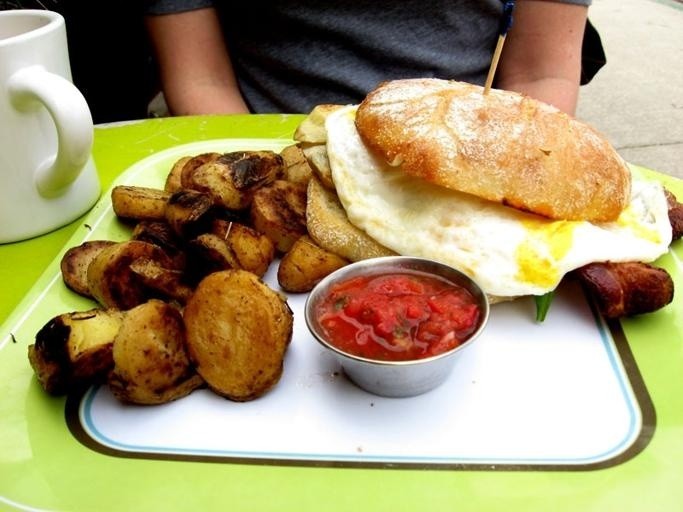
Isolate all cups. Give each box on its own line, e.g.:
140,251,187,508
1,8,103,246
302,255,489,398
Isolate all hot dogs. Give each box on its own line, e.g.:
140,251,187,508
293,76,683,320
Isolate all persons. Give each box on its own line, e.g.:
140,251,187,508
141,1,590,118
